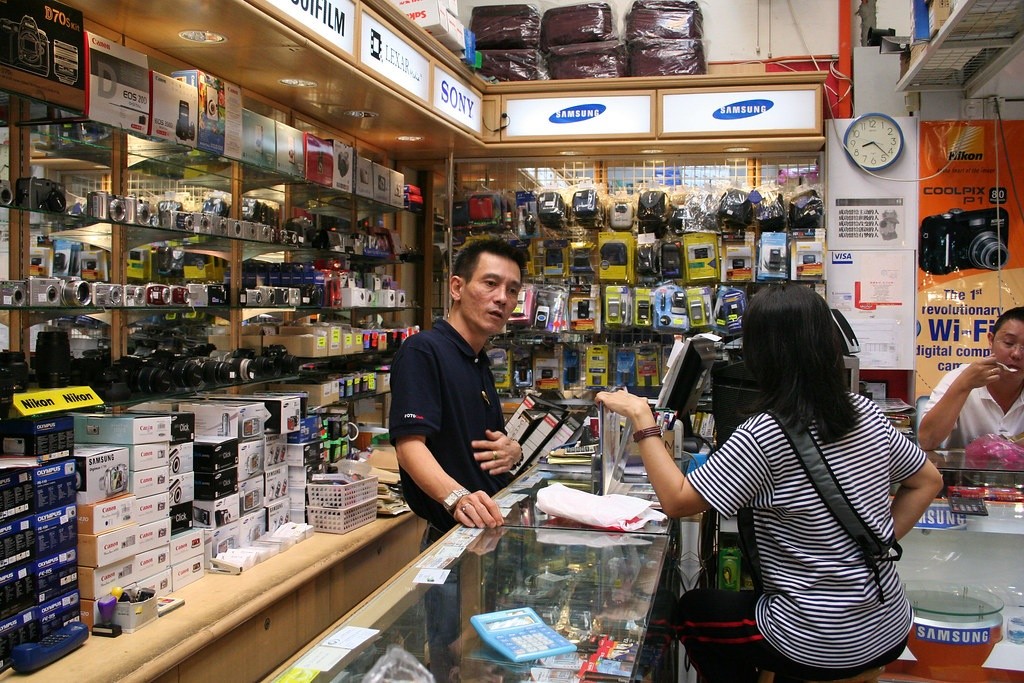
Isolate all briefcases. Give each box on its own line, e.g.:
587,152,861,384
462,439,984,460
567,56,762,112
469,3,542,80
626,0,706,77
542,2,627,78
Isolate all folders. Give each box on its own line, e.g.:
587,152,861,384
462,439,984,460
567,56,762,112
505,393,584,479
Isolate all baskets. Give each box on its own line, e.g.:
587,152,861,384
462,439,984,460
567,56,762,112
305,473,379,535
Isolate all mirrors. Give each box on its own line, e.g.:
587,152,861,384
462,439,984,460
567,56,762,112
0,143,314,350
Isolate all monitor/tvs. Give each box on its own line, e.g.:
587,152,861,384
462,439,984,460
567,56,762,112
662,336,718,454
713,352,859,451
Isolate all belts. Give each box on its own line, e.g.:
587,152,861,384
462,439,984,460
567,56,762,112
425,524,444,542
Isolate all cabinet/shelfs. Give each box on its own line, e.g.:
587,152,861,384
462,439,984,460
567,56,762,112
451,82,825,424
263,455,691,683
0,0,487,683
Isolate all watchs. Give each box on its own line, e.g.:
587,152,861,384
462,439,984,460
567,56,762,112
442,488,472,516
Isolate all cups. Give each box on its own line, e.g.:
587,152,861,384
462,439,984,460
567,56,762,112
661,430,675,461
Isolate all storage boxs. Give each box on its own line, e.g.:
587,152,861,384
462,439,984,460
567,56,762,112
341,286,405,308
171,69,425,212
209,324,262,355
391,0,482,72
899,0,950,78
263,321,419,358
0,370,391,675
717,544,742,591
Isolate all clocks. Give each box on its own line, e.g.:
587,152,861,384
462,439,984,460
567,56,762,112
843,113,904,172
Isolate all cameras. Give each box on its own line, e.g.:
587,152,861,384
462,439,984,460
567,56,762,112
176,100,195,142
361,168,370,185
206,87,218,121
287,136,296,165
98,463,129,495
377,175,387,192
214,509,232,527
919,206,1010,275
192,507,211,525
253,125,264,155
0,175,328,403
86,425,99,435
3,437,24,454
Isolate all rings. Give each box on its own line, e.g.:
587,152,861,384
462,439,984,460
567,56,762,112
463,508,466,513
990,370,995,375
492,450,498,460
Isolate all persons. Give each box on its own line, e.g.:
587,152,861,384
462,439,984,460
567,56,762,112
592,285,944,683
388,235,525,683
917,307,1024,489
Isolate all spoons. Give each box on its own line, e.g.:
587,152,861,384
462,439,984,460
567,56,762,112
996,361,1018,373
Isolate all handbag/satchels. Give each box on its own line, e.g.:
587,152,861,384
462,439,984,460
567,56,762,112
717,188,755,231
637,190,670,240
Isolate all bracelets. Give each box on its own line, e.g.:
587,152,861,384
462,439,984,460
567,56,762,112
508,437,524,470
633,425,662,443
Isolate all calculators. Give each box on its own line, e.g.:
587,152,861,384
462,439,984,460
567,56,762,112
470,607,577,661
948,495,989,515
